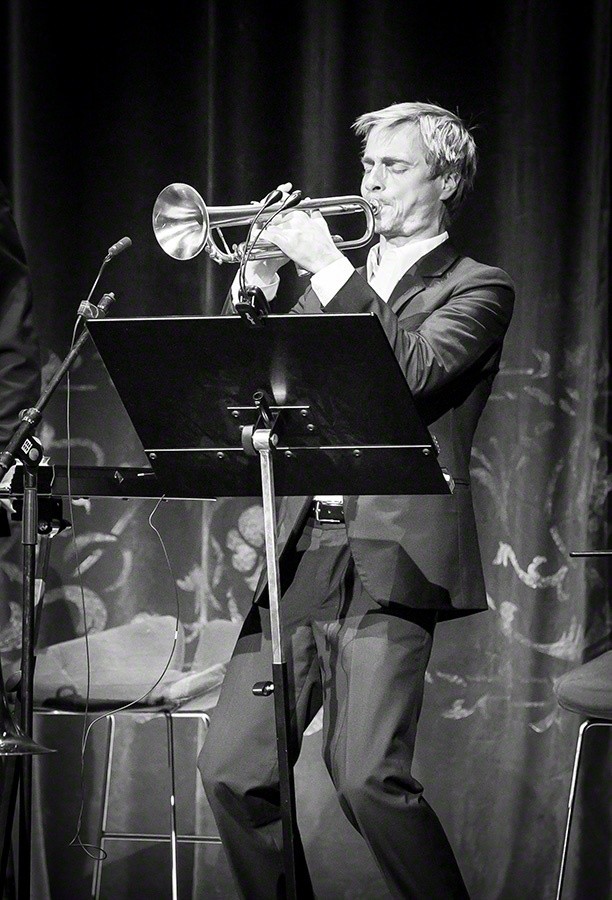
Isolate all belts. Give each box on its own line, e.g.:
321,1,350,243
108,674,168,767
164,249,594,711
306,500,347,523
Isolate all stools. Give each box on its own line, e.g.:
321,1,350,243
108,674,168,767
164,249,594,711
33,617,244,900
554,649,612,900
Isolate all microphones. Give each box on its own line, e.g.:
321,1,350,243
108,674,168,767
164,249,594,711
108,236,132,257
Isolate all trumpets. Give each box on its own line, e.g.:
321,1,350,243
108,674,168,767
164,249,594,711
152,182,383,266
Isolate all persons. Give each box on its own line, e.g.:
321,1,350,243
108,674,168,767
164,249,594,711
1,255,42,451
196,102,517,900
